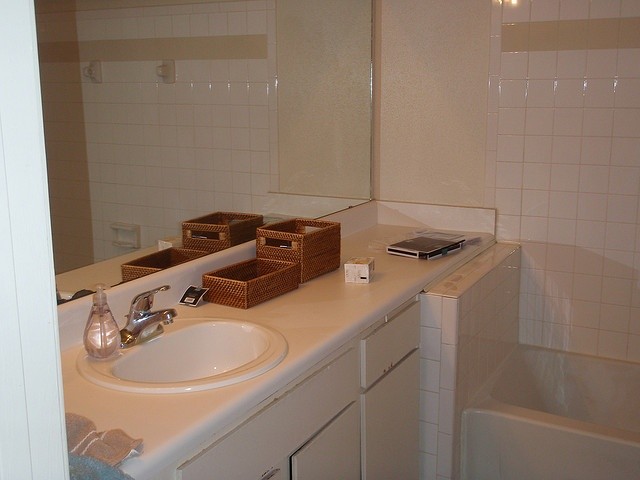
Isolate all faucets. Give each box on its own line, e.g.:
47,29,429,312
121,284,177,348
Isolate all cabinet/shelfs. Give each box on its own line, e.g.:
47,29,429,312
358,296,421,480
176,345,359,480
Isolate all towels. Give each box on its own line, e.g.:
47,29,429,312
68,455,135,480
66,413,143,468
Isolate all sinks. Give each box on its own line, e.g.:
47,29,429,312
111,321,271,383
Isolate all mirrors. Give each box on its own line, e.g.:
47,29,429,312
34,0,373,304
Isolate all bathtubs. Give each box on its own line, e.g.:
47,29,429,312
461,342,640,480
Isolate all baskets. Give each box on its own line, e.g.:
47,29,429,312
181,211,265,252
203,258,301,310
121,247,208,283
255,218,341,282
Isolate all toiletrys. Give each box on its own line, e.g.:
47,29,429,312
83,283,121,360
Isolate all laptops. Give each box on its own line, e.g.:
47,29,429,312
387,237,461,260
